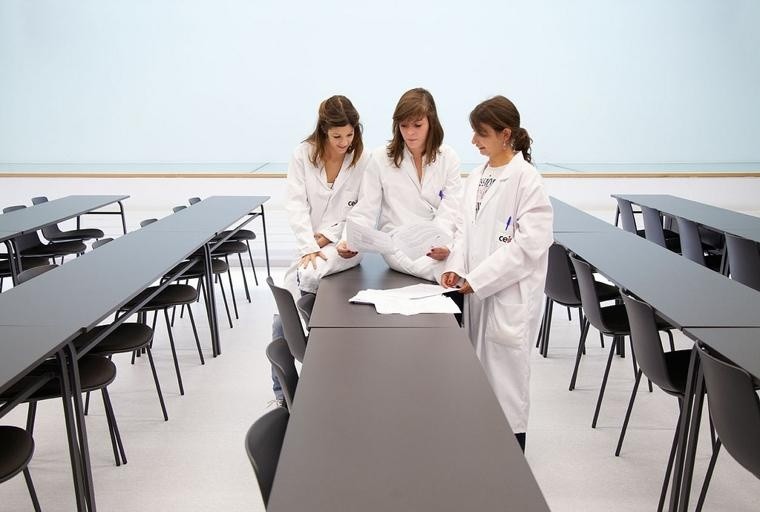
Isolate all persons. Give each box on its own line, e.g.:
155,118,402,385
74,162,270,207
336,88,468,327
442,96,555,455
269,95,371,410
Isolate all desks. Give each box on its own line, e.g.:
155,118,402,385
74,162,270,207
550,196,760,512
265,250,553,512
2,195,272,437
1,188,131,241
608,194,760,244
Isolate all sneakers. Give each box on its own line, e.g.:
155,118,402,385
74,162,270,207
265,399,287,409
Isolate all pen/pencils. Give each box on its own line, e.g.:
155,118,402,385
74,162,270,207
438,190,443,202
503,215,512,232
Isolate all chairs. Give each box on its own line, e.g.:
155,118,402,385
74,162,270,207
567,252,676,429
3,204,86,276
266,276,309,363
11,263,170,424
540,242,608,358
265,336,299,413
90,237,205,396
674,216,705,267
0,194,270,512
640,205,667,249
725,232,760,292
616,197,638,235
297,293,316,325
695,339,760,497
170,206,253,303
244,406,291,511
614,285,702,510
30,196,105,259
188,196,272,279
138,218,239,329
